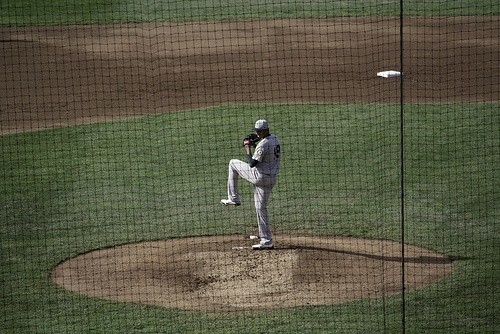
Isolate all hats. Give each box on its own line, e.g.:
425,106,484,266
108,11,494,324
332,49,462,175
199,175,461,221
251,119,268,130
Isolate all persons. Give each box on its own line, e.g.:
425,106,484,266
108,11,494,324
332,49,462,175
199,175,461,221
220,119,280,249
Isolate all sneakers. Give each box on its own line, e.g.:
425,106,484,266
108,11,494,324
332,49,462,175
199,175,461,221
252,244,274,250
220,199,241,206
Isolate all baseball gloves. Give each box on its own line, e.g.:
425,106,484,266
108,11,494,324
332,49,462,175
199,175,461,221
242,133,260,147
